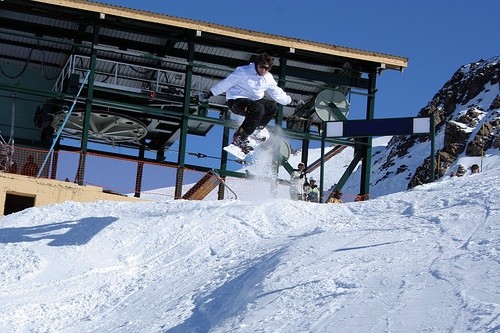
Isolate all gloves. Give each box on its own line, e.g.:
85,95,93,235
287,98,305,107
198,90,213,102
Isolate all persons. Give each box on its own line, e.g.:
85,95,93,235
450,171,456,176
327,188,343,203
0,150,18,172
471,163,480,173
198,51,307,155
310,176,319,202
20,155,41,176
302,181,311,201
354,192,366,201
289,161,306,201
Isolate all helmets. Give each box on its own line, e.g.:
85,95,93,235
309,176,317,183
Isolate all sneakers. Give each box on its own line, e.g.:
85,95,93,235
251,128,266,142
233,130,254,153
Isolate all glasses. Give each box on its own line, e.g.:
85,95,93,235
259,64,271,69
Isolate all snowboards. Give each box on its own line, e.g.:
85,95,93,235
223,127,270,161
